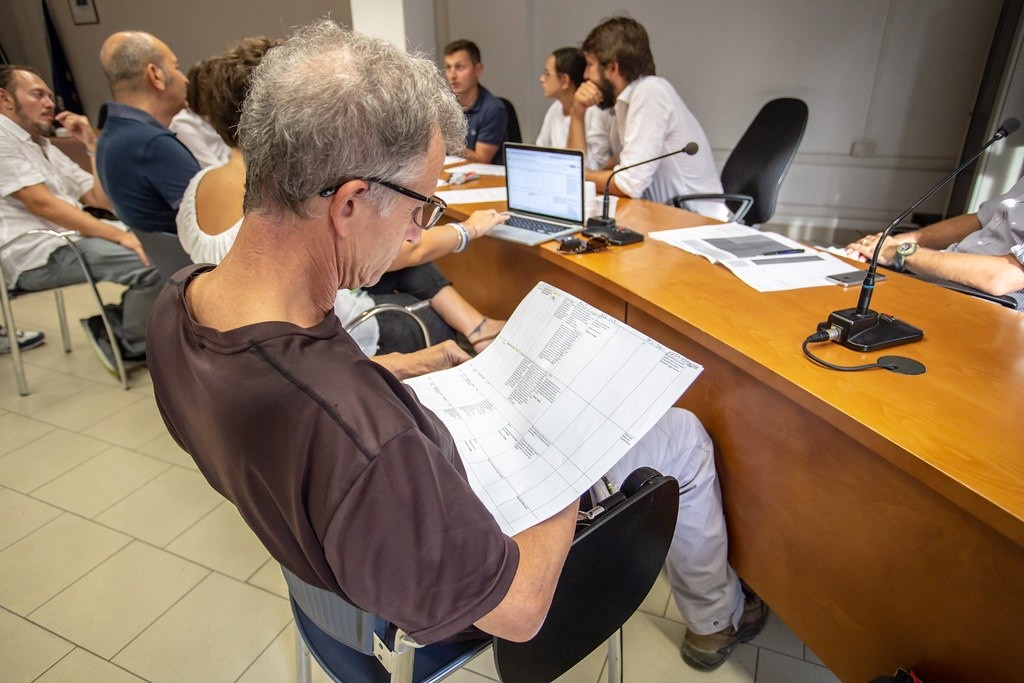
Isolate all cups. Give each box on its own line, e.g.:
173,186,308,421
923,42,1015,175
585,181,596,220
595,195,619,220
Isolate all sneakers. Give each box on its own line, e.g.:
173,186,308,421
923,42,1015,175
79,315,122,376
0,324,45,353
681,576,770,671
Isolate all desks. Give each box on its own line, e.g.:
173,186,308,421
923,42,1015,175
429,149,1024,683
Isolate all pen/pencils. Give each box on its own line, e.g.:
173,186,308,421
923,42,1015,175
764,249,805,255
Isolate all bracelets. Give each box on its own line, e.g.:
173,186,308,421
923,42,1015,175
86,151,96,156
446,222,469,253
467,222,478,238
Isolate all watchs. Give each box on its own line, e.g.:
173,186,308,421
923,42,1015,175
895,242,917,272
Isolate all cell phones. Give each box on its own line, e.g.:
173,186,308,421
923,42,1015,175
826,270,885,288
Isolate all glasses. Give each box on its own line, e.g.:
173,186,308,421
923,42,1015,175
555,235,611,254
537,71,562,77
321,177,449,231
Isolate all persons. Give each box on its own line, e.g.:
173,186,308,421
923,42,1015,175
95,31,203,234
534,47,601,171
0,64,190,376
145,23,764,669
174,38,510,356
443,40,508,163
169,63,232,169
843,177,1024,322
567,16,730,223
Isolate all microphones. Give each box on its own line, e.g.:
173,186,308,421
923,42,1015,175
817,117,1022,353
583,142,698,246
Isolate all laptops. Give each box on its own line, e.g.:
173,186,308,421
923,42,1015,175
483,143,586,246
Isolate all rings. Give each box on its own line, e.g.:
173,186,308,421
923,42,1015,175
491,212,495,216
862,239,866,244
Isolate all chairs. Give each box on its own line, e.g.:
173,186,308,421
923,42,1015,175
673,96,809,227
134,228,435,352
279,470,625,683
0,203,137,397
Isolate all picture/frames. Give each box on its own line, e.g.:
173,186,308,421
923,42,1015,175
67,0,101,26
